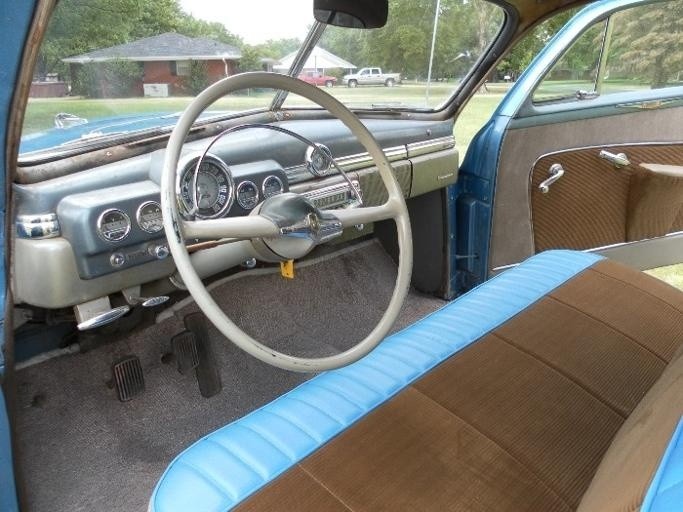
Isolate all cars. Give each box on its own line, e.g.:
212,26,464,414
297,70,337,88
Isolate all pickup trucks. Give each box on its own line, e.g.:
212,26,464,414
342,67,402,88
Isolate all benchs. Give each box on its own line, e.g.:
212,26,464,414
148,248,682,511
